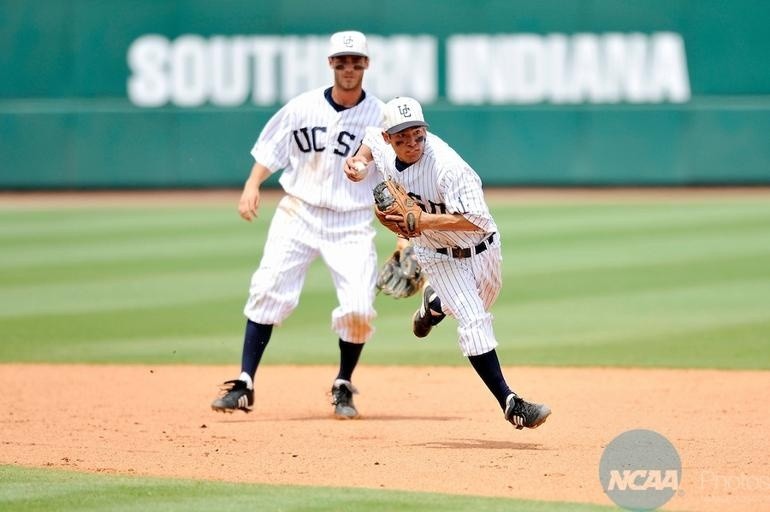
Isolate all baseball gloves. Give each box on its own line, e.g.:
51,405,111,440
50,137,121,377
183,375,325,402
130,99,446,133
376,238,426,299
372,175,422,240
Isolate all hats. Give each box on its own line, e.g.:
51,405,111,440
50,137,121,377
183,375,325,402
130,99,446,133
327,30,369,58
380,96,429,134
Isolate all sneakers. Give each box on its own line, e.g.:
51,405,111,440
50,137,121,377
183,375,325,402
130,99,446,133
327,379,359,416
504,394,552,430
412,280,447,339
211,380,254,413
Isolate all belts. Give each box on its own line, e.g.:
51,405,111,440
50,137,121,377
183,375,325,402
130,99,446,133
436,233,496,258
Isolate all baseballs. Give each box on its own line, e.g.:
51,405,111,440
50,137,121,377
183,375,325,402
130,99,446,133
351,162,369,180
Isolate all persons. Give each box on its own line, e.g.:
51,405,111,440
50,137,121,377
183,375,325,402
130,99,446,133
211,30,551,427
